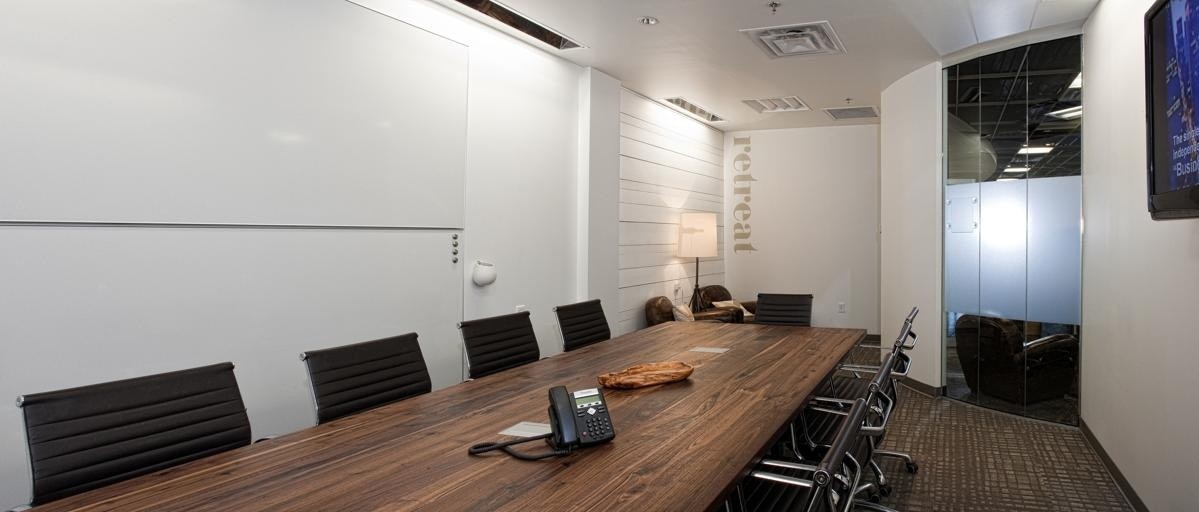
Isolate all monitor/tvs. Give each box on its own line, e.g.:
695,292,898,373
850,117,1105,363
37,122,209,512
1144,0,1199,220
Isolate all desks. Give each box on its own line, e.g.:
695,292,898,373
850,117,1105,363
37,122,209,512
15,318,870,511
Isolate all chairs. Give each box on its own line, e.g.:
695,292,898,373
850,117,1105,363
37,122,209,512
646,285,815,328
954,312,1079,408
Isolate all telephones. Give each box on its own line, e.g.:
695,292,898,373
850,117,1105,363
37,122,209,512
548,386,616,449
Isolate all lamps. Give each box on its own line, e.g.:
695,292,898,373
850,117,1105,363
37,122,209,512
676,212,719,312
